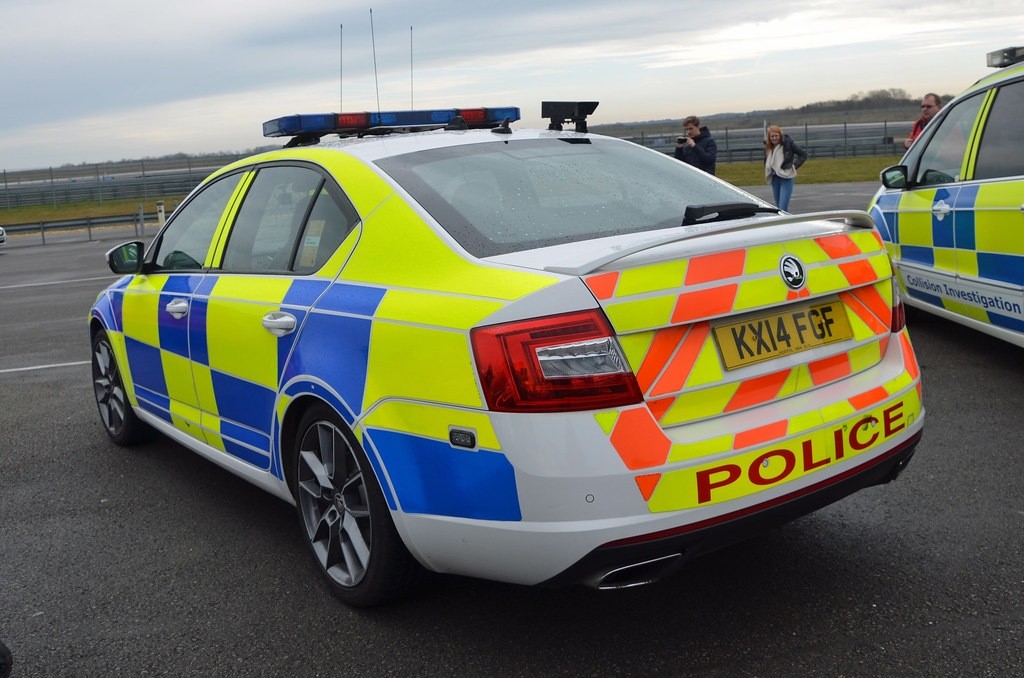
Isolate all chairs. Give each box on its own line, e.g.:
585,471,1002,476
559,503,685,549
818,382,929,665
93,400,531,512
454,183,501,225
272,193,330,271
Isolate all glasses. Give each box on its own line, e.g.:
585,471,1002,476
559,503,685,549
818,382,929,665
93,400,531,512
921,104,937,109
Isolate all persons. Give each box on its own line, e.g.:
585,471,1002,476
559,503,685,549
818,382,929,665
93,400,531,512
904,93,966,171
764,126,807,211
675,116,717,176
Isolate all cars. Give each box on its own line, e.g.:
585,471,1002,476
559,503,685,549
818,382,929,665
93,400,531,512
86,100,925,614
865,43,1024,348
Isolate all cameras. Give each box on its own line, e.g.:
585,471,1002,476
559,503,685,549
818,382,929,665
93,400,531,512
678,137,687,143
